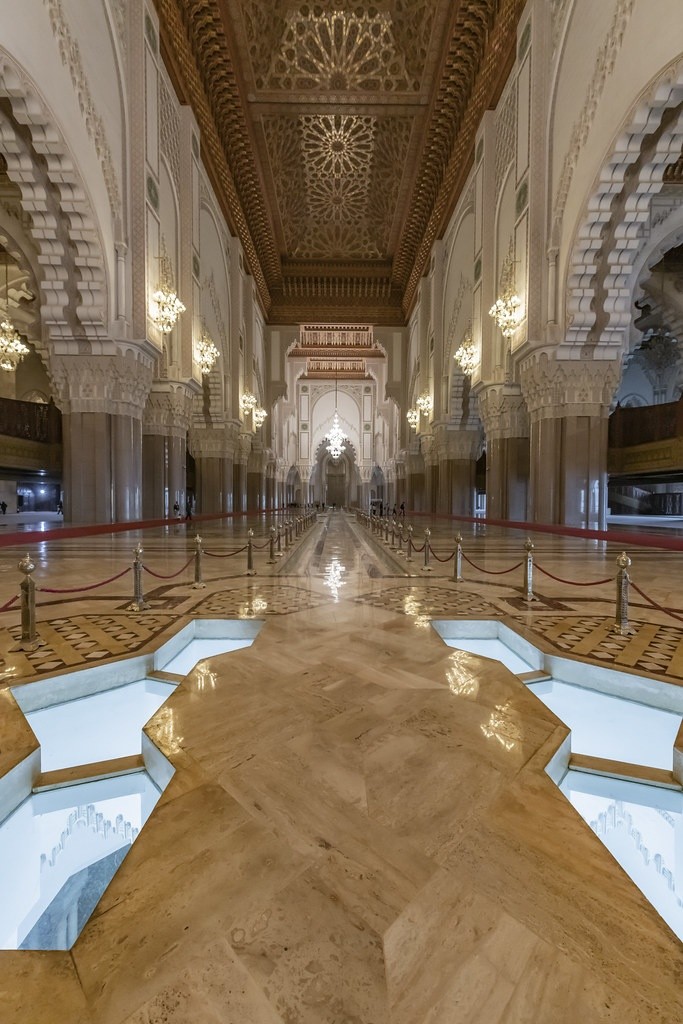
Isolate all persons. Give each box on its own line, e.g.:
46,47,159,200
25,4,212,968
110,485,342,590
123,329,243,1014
379,502,383,516
392,504,397,515
1,501,8,514
56,501,63,515
174,501,179,516
399,502,405,516
322,503,325,511
185,501,192,520
384,503,389,515
372,504,376,514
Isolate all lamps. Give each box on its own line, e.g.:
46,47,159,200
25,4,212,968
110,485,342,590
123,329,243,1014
196,321,221,376
152,254,186,335
489,282,518,340
407,406,418,431
0,250,30,372
452,316,478,378
325,359,347,460
416,389,432,417
241,383,268,428
